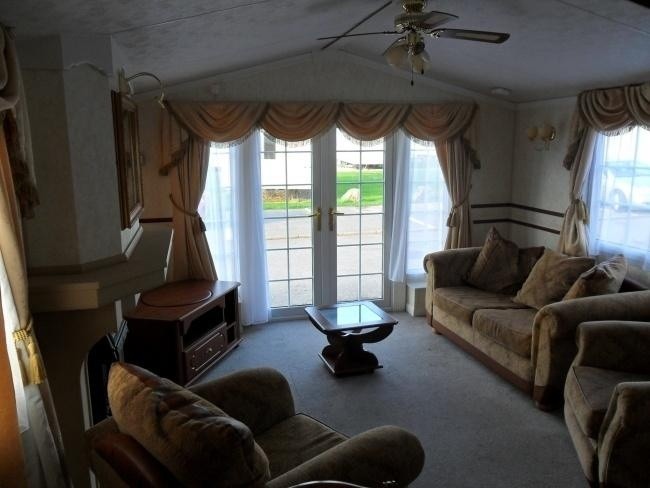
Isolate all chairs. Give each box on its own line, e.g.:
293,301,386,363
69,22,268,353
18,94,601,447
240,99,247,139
82,366,428,487
561,321,649,487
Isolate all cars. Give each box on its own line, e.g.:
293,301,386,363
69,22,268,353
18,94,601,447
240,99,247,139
601,158,650,213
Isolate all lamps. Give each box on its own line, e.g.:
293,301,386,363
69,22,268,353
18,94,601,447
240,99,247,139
387,0,432,86
524,121,560,153
119,65,167,109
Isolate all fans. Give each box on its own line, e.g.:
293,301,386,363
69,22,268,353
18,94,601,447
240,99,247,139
316,1,510,57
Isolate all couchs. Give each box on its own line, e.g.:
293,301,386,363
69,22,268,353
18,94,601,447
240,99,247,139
423,245,649,409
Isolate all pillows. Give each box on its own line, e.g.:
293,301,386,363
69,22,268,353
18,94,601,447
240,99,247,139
560,251,628,301
467,228,544,297
106,355,272,487
512,244,595,312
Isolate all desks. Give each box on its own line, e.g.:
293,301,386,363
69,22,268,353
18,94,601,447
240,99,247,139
124,278,243,391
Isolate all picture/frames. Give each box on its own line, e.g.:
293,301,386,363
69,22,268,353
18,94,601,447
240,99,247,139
111,88,146,230
